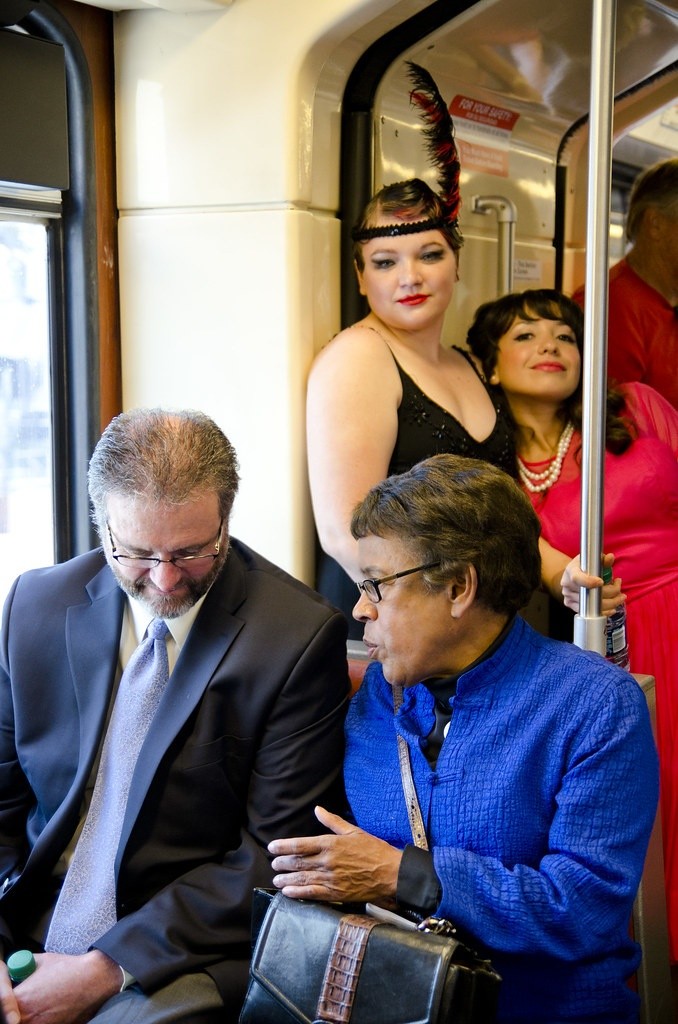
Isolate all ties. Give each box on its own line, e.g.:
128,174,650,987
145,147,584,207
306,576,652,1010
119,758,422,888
45,617,169,956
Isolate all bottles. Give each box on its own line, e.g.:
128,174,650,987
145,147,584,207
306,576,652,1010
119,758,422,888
602,567,630,675
6,949,36,987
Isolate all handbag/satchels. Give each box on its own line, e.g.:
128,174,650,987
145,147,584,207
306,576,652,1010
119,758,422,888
239,888,504,1024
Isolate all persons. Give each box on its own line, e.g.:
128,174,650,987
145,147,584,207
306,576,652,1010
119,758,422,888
305,179,515,644
1,408,353,1024
267,454,659,1024
465,288,678,967
570,156,677,414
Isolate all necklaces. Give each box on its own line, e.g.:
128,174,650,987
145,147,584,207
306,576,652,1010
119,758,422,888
514,419,576,493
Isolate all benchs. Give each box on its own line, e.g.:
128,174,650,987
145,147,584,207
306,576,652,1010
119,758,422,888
339,642,675,1024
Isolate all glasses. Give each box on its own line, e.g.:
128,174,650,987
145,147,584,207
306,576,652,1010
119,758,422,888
356,558,481,603
105,515,223,568
